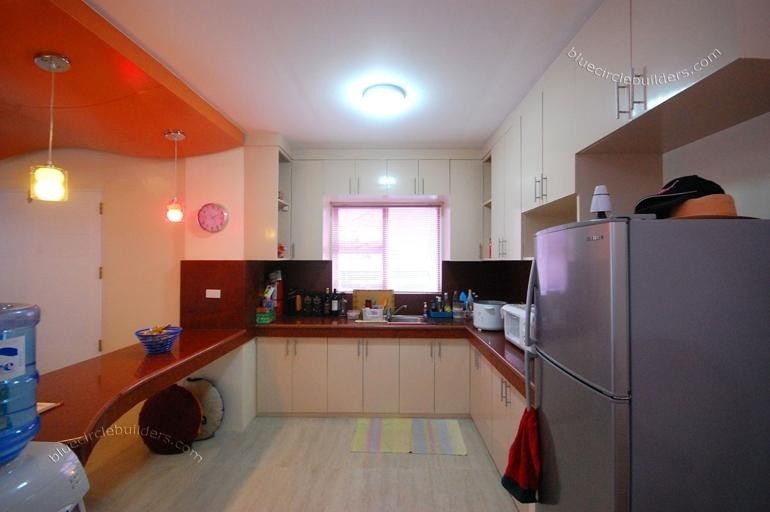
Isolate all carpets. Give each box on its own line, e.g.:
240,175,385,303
350,416,469,455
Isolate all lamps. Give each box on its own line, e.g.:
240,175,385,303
27,54,71,205
162,130,187,222
362,83,407,112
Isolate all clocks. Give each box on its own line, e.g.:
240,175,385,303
199,203,228,232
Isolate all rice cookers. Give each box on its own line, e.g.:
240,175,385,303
473,300,508,331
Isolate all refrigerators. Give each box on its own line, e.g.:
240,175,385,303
533,216,770,512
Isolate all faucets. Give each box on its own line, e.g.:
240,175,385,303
386,304,407,321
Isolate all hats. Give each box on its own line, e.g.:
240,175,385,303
634,174,725,219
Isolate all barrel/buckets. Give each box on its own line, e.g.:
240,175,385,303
0,302,41,467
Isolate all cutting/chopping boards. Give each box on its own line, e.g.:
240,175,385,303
351,289,395,315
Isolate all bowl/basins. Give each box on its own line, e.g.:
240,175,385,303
135,326,184,355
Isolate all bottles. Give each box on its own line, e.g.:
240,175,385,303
424,289,473,320
284,285,348,317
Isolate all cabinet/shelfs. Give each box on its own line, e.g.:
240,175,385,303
483,152,491,261
332,159,386,203
489,126,574,259
493,364,537,511
522,74,662,223
386,160,449,202
441,159,483,260
400,338,471,416
469,342,493,451
243,144,294,259
256,337,328,416
292,160,332,261
569,0,770,156
328,339,399,416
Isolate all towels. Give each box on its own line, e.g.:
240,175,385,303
500,405,544,504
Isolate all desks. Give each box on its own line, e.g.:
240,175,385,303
32,329,258,466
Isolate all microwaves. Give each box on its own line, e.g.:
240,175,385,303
502,303,538,356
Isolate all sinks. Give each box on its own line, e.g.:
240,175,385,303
385,316,429,322
